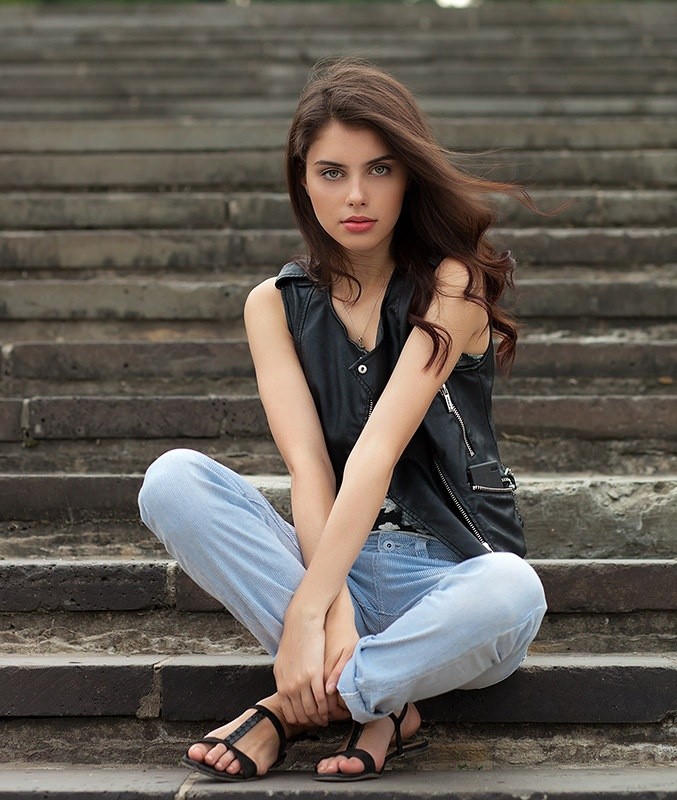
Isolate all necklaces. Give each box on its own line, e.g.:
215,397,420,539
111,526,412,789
331,265,394,351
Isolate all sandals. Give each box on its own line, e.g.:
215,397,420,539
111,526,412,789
179,705,289,782
313,692,429,782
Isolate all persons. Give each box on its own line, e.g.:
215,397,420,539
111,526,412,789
139,55,548,783
